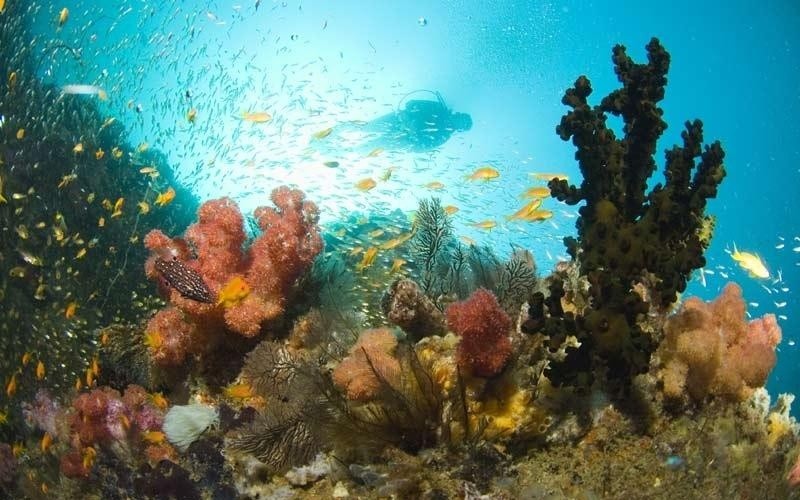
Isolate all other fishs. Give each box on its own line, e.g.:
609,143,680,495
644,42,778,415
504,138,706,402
0,0,580,496
699,235,800,353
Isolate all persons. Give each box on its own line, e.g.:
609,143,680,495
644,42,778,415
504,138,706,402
307,87,473,159
